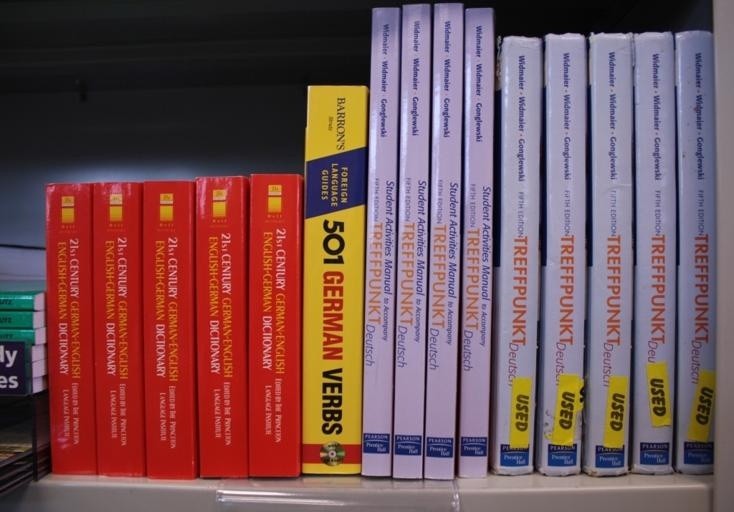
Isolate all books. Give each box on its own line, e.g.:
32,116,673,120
536,32,587,476
46,182,97,474
141,181,198,479
0,285,50,499
244,173,301,478
581,33,633,476
672,31,714,474
195,176,248,479
91,180,147,479
302,85,368,476
629,33,676,475
390,3,431,480
360,6,400,478
423,3,463,479
459,8,493,480
493,36,542,475
